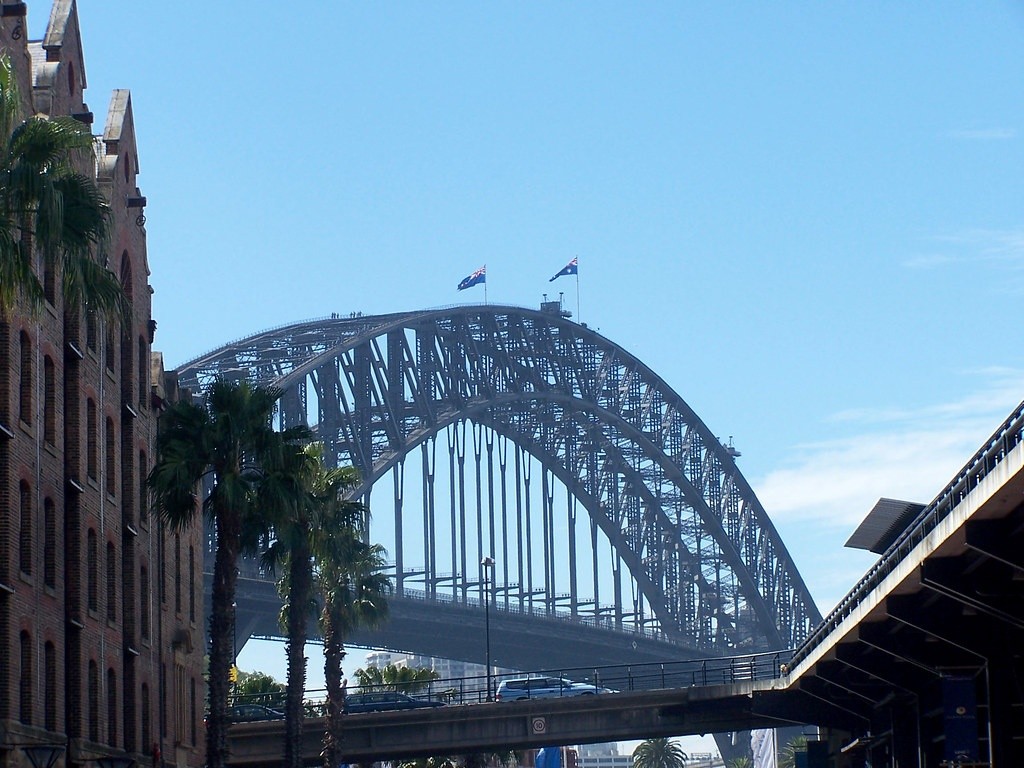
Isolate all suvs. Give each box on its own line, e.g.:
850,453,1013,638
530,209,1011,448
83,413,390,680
341,691,448,712
494,678,611,701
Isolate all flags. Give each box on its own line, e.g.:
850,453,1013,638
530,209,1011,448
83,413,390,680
549,256,577,282
457,265,486,291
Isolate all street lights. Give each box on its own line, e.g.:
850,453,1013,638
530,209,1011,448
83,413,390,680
482,558,497,701
234,602,239,707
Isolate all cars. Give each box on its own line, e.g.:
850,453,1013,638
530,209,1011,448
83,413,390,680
229,706,285,723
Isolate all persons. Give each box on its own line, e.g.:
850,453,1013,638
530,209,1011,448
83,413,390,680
331,310,362,319
